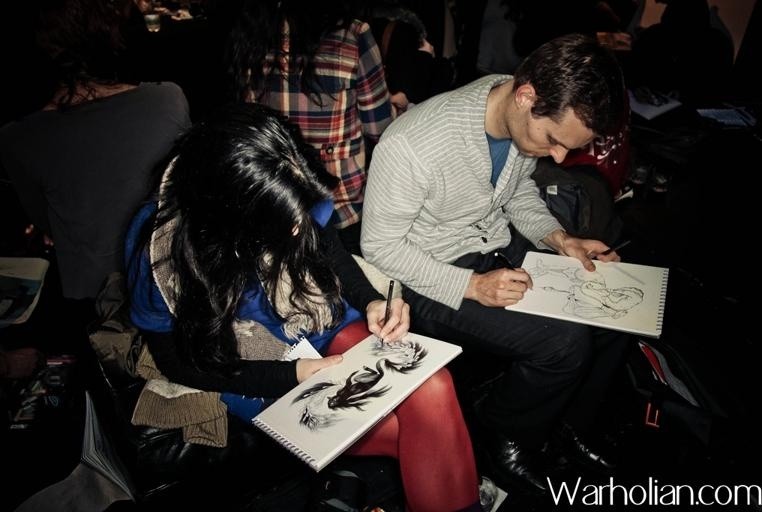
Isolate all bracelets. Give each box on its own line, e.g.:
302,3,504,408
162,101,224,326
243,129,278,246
555,234,571,252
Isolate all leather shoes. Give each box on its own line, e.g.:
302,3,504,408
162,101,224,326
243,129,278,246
501,439,556,492
556,418,618,469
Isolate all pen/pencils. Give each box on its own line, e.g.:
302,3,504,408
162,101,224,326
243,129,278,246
592,240,632,260
499,252,533,291
380,281,395,350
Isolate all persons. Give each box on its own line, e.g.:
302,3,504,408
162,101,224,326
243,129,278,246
624,0,702,195
23,10,194,363
213,2,408,257
118,102,486,511
360,32,631,499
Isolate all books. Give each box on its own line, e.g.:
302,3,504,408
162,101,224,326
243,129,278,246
248,328,465,473
503,251,670,340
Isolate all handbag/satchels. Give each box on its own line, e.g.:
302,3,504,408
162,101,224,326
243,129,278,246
246,464,376,512
